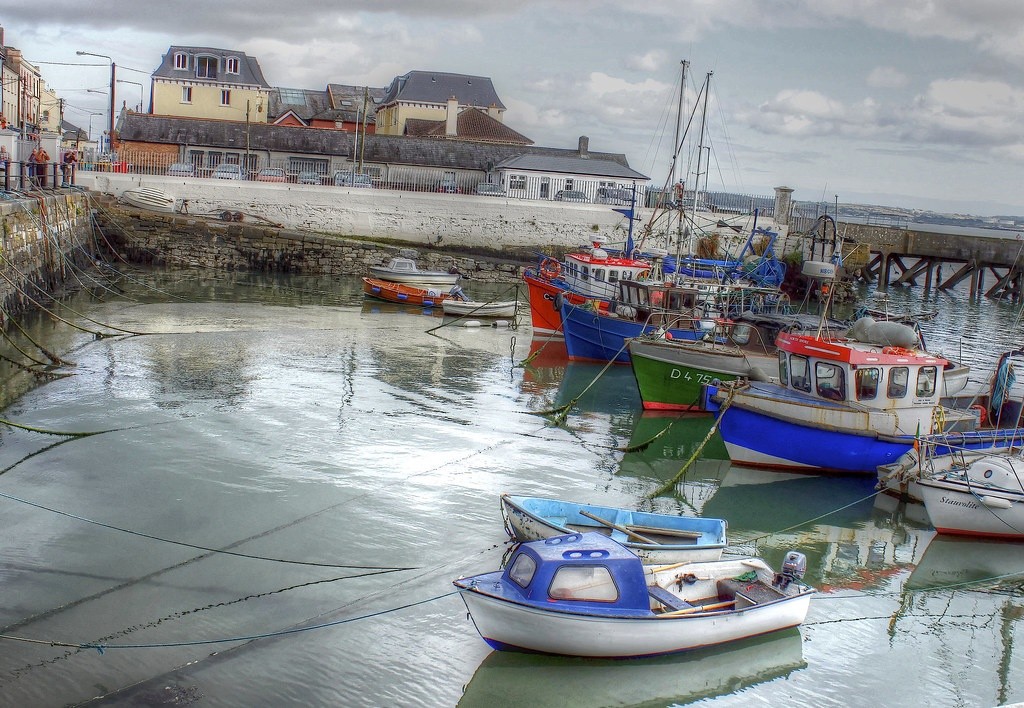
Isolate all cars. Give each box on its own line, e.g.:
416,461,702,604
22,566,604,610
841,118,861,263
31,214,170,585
429,180,461,193
680,197,713,213
211,164,248,181
98,155,111,163
297,171,321,185
554,190,589,203
255,168,289,183
471,183,507,197
334,172,372,188
166,164,196,177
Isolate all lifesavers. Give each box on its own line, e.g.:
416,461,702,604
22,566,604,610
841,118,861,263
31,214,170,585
595,308,619,318
540,257,561,279
882,346,917,358
969,405,987,423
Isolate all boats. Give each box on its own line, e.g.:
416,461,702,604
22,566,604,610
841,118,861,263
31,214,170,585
912,435,1024,539
452,530,817,658
455,626,808,708
552,361,644,414
613,409,731,482
362,257,522,317
699,460,917,594
698,277,1024,475
900,533,1024,591
500,493,728,568
122,187,176,213
360,296,444,319
522,235,790,335
623,291,970,411
552,279,926,364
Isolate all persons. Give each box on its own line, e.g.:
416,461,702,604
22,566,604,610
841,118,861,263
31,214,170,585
0,144,12,171
64,148,78,186
28,147,50,188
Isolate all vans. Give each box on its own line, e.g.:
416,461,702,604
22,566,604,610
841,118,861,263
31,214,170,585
594,188,636,206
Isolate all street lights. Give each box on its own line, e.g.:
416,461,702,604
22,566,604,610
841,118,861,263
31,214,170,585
77,51,115,150
87,89,108,95
89,112,102,141
235,129,249,175
117,80,143,113
352,105,361,186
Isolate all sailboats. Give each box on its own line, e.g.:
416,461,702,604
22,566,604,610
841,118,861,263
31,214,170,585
601,60,788,290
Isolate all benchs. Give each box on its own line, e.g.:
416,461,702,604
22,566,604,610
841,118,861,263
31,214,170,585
647,584,704,615
